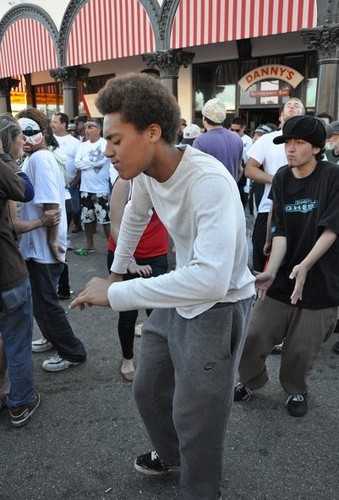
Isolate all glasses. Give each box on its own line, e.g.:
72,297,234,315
230,128,241,133
84,122,100,129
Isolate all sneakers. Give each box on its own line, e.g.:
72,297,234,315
41,351,81,372
8,389,41,427
133,450,183,476
32,337,55,352
0,392,10,412
232,375,271,401
284,390,312,417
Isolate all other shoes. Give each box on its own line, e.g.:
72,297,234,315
331,341,339,354
67,239,74,250
93,229,97,233
57,289,70,299
133,323,143,337
120,359,136,382
270,341,284,355
71,227,82,233
74,246,97,255
333,319,339,333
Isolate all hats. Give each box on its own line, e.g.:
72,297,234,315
182,124,203,139
69,115,88,123
201,98,227,123
272,116,327,146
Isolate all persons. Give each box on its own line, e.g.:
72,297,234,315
67,74,256,500
0,77,339,427
